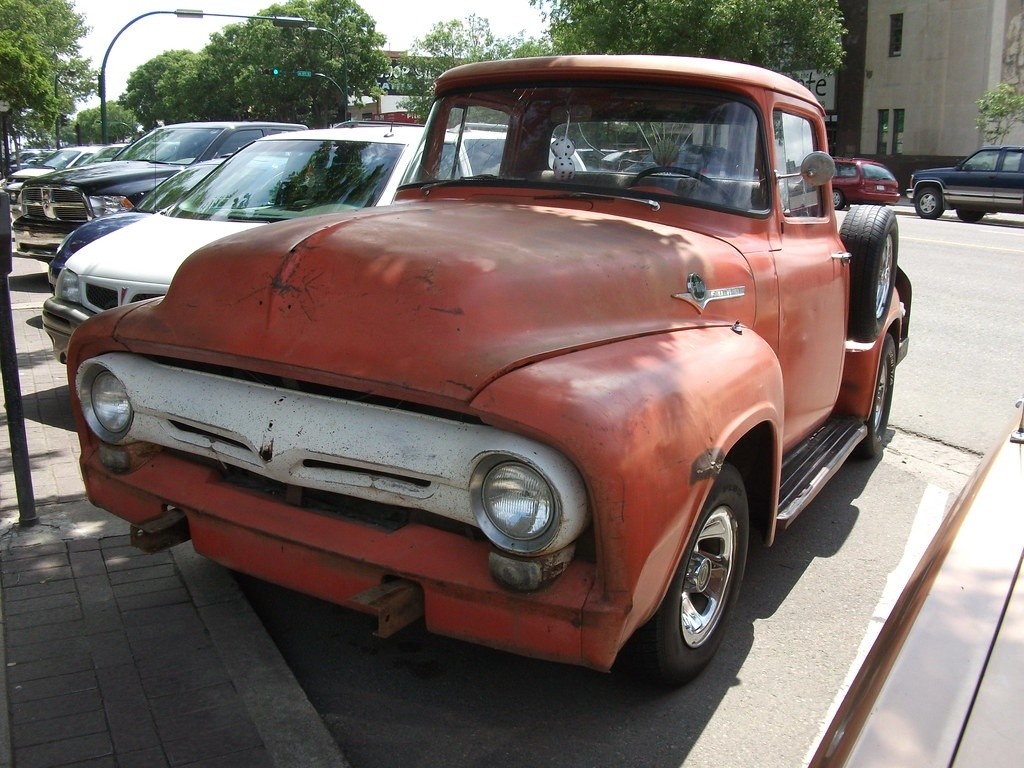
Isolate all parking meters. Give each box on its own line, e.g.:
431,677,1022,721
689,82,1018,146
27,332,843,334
0,190,39,527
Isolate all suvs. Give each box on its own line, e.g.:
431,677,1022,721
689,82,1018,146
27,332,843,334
12,122,316,260
828,157,900,210
907,145,1024,223
62,54,915,699
36,120,591,365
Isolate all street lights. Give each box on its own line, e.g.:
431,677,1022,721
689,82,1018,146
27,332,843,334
55,68,76,150
95,9,308,148
307,24,352,122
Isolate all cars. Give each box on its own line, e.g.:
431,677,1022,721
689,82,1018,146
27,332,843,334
45,153,291,295
6,144,129,204
575,145,755,181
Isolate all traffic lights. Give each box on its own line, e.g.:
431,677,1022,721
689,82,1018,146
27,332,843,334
262,67,279,77
60,113,68,127
